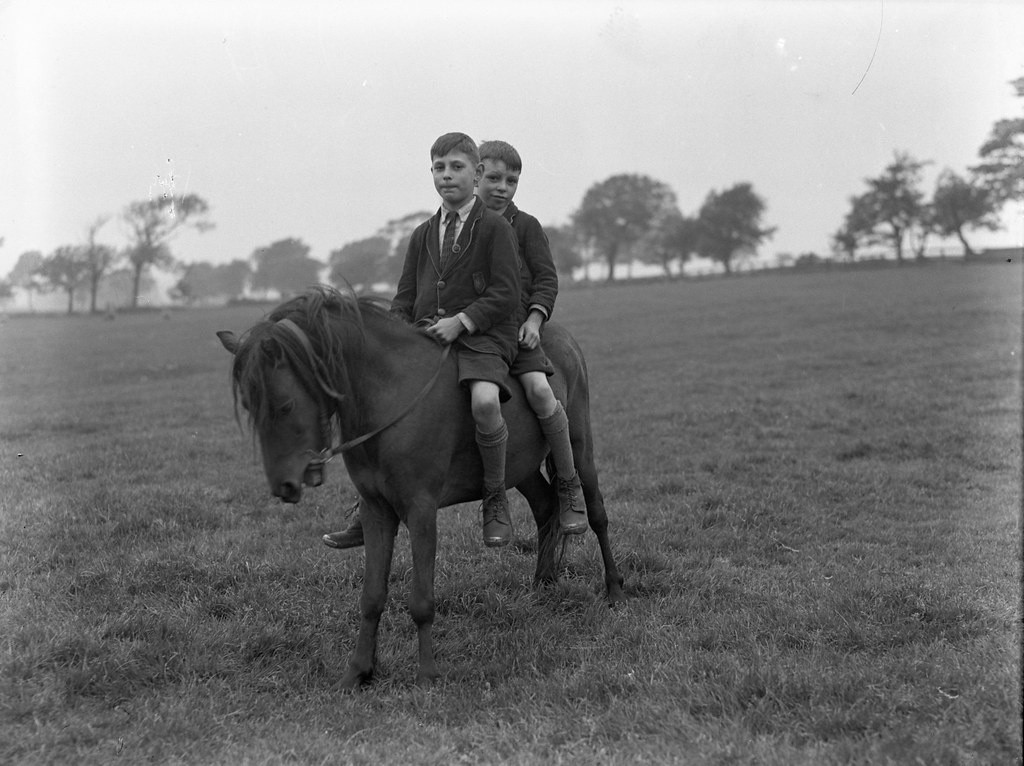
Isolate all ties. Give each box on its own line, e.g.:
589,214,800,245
440,211,458,271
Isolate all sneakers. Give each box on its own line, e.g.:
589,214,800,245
558,469,589,534
478,482,514,546
322,503,364,548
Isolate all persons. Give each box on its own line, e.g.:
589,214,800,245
473,140,589,534
322,133,526,550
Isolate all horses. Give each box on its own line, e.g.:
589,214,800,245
213,286,628,692
